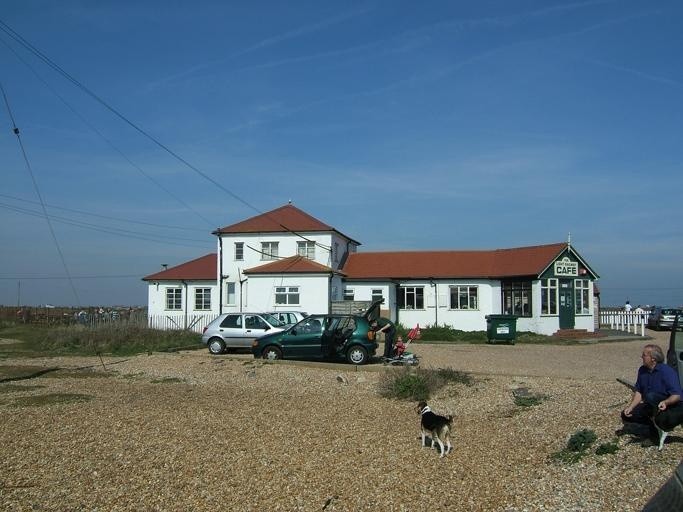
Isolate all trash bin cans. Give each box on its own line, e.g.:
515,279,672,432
485,315,518,345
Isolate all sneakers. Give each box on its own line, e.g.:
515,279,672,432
642,434,659,447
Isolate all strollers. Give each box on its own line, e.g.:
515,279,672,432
378,322,420,364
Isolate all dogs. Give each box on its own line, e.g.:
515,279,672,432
413,399,453,459
638,400,683,451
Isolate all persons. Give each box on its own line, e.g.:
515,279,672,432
624,301,631,311
369,317,395,359
619,343,681,448
634,305,643,312
394,335,404,358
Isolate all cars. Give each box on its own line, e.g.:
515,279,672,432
250,311,309,329
251,298,385,365
201,311,289,355
646,307,682,330
665,314,682,391
